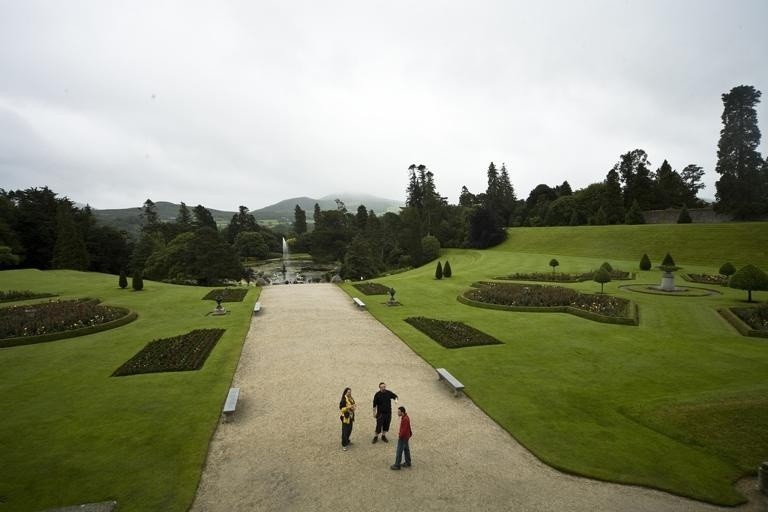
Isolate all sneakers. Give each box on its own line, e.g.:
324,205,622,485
342,441,354,451
372,436,388,444
390,462,411,471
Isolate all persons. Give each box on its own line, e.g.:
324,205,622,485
371,382,400,444
391,406,412,470
339,387,357,450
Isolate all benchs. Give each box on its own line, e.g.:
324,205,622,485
253,301,262,313
222,387,240,417
435,368,465,397
352,297,367,311
